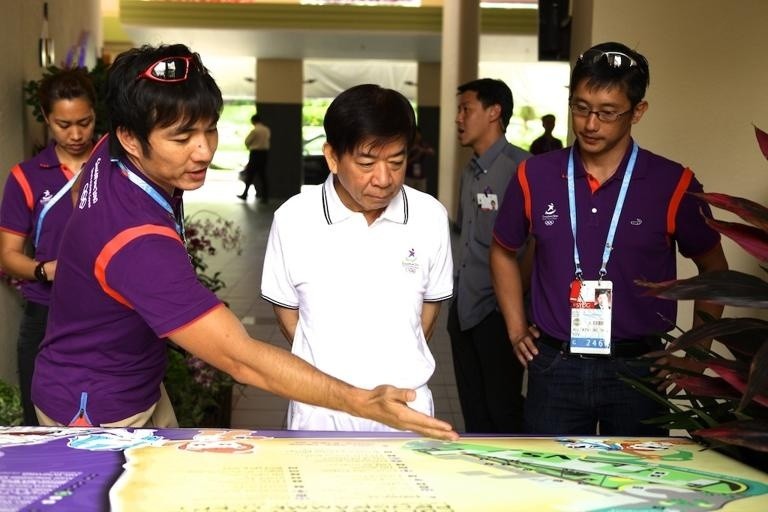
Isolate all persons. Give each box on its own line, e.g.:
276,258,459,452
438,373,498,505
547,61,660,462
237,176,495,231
404,123,436,194
528,115,564,155
258,82,455,431
443,77,537,434
593,288,611,310
0,65,102,427
486,39,733,437
234,114,273,204
26,39,464,449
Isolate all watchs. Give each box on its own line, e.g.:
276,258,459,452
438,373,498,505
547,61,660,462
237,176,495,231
33,259,49,287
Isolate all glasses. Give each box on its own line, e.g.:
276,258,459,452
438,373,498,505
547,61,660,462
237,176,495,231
121,52,205,95
568,96,630,123
579,48,639,71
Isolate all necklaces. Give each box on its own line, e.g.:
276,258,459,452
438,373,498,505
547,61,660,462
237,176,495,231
597,167,616,184
